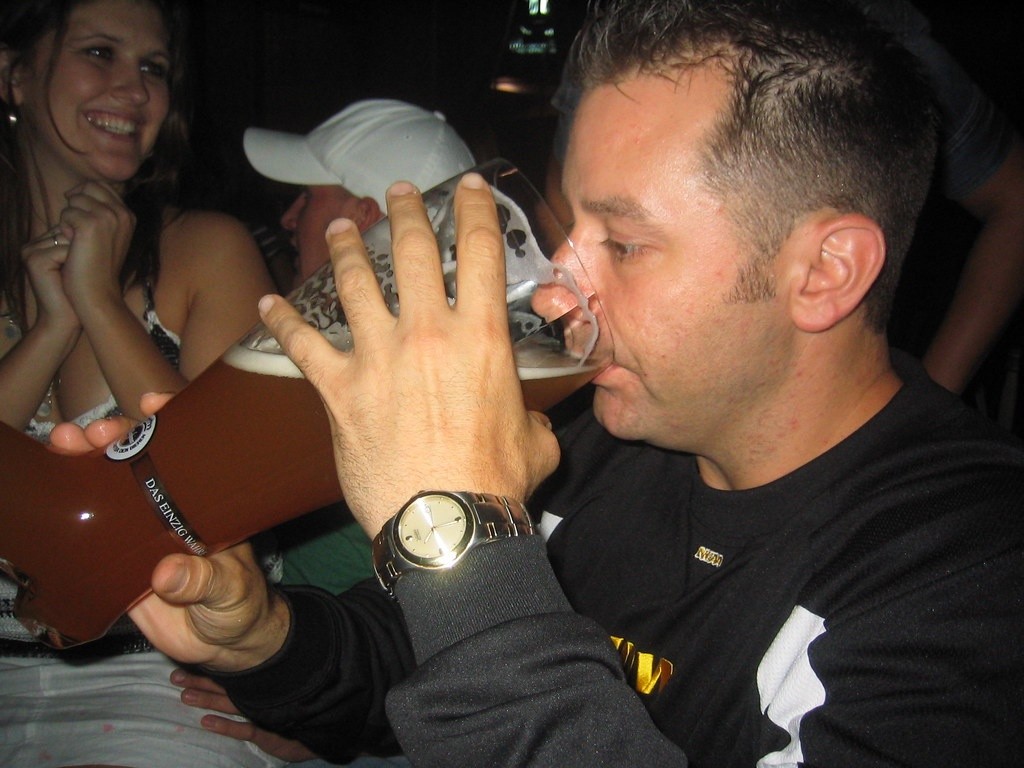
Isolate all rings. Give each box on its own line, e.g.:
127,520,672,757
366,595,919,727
53,237,57,246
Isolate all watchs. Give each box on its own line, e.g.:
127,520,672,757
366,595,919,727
371,489,537,596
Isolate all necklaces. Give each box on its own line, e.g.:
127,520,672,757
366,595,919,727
5,316,62,417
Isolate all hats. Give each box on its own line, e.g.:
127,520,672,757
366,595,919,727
243,95,476,248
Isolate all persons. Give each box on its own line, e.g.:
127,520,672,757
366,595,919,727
0,0,1024,768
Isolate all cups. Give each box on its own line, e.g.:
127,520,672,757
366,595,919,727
0,158,616,650
